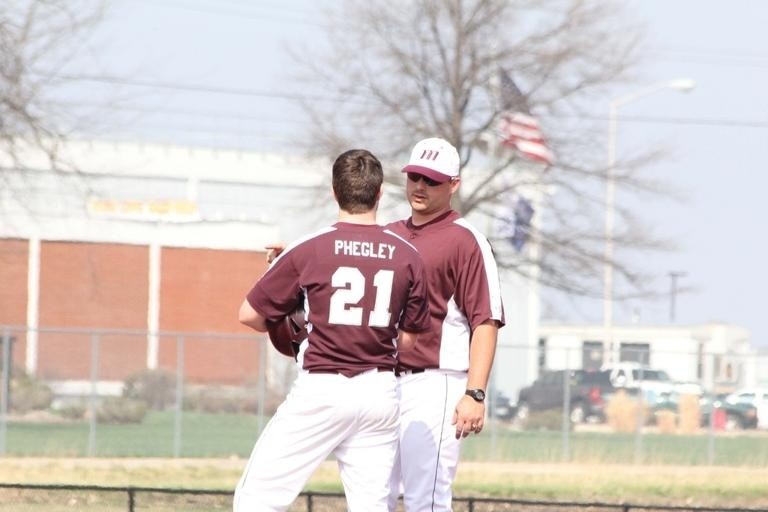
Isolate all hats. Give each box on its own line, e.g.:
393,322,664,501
401,136,461,181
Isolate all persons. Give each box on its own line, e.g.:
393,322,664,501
232,138,506,512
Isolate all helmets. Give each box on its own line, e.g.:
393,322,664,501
266,305,308,363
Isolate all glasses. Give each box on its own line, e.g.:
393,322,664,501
407,171,442,186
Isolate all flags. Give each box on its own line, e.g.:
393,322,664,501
498,67,554,166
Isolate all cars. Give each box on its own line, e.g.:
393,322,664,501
520,369,767,432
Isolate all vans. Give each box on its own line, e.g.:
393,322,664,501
600,361,673,396
